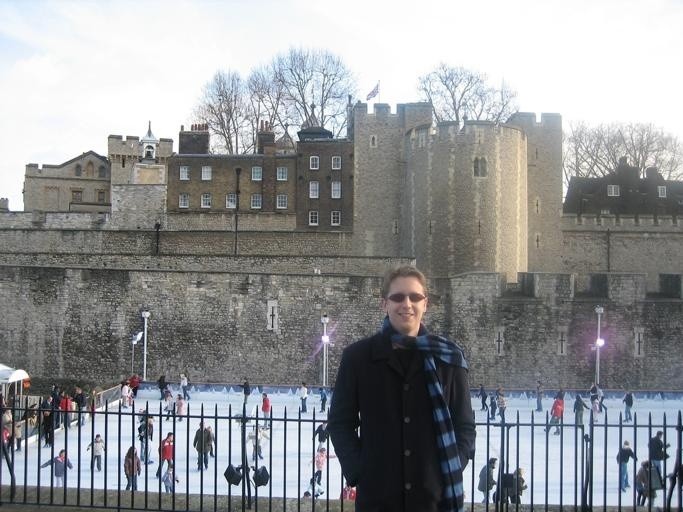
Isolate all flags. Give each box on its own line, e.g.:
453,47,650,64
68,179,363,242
366,84,378,101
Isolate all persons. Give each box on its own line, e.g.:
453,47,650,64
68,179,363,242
536,378,634,435
478,383,506,422
300,380,356,499
616,431,671,506
325,264,475,510
0,372,270,493
478,457,528,505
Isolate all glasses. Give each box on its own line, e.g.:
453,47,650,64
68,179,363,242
386,291,424,304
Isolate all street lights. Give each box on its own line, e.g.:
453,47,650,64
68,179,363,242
594,306,604,386
320,312,330,387
141,310,151,381
131,331,144,378
